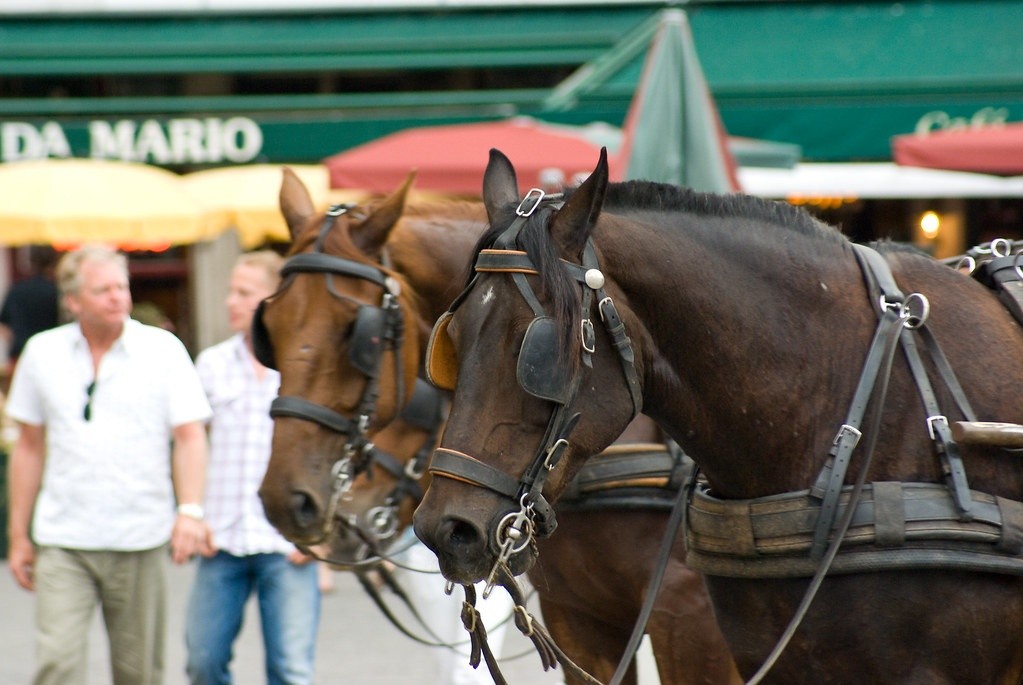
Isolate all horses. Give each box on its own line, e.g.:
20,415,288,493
255,165,745,685
411,144,1023,685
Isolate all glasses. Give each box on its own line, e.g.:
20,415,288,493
84,382,95,420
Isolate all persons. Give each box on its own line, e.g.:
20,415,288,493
1,246,328,684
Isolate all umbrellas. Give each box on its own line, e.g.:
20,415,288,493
1,119,802,256
615,8,742,196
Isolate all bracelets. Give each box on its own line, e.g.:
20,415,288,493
179,504,207,519
